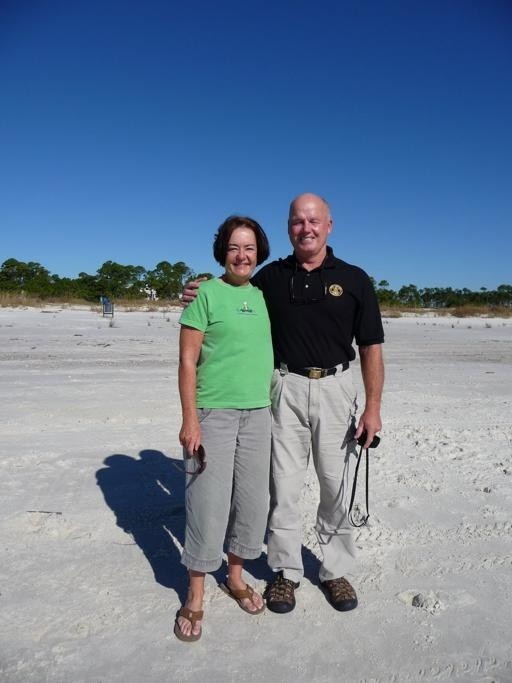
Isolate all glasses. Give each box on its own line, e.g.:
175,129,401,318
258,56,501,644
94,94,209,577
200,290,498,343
291,276,327,303
171,444,206,474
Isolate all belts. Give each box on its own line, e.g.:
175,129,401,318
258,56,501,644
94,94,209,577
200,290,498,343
275,361,349,379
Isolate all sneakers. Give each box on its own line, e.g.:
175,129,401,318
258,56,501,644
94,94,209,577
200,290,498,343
266,571,299,613
319,576,357,611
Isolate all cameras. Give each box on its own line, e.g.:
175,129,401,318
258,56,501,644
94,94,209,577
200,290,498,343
357,432,380,448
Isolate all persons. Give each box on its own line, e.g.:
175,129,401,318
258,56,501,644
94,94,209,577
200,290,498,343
173,217,277,643
181,192,386,615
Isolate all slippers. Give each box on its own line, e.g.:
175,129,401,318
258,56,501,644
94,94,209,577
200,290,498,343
174,607,203,642
220,580,265,615
